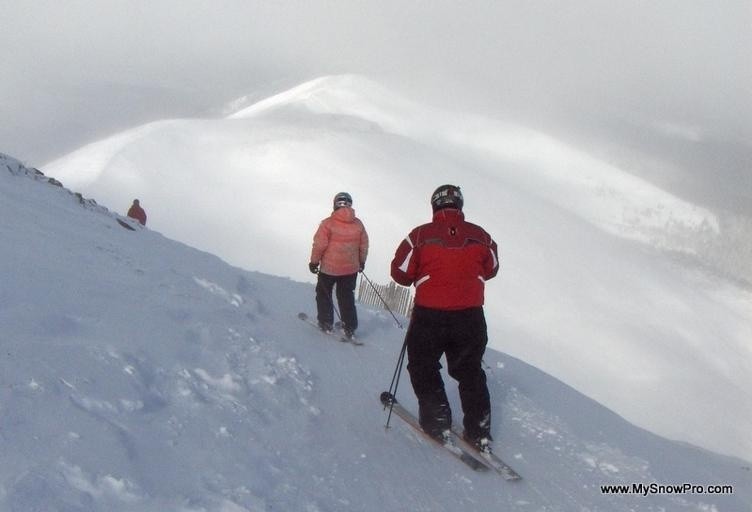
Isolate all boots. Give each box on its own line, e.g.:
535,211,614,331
316,295,334,333
407,366,452,449
337,295,360,334
458,369,492,452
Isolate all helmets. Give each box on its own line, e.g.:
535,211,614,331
334,192,353,210
431,184,464,211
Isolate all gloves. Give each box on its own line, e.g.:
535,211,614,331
358,263,365,273
309,261,321,273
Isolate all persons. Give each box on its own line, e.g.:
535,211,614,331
309,191,369,335
128,199,147,226
388,183,501,454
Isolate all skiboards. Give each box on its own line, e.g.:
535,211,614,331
380,392,520,481
299,313,365,344
